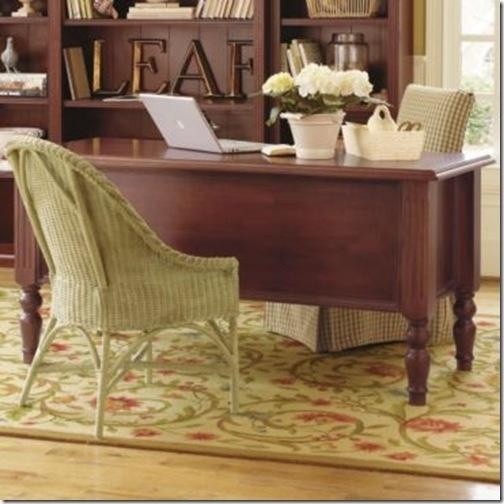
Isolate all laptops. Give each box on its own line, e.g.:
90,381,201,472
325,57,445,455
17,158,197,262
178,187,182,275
138,93,269,154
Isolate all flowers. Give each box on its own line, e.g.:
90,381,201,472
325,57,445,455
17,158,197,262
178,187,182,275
258,59,381,118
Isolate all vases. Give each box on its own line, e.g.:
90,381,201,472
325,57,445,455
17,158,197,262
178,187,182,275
281,110,347,156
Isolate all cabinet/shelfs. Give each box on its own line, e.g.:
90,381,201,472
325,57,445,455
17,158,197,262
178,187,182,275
0,0,411,259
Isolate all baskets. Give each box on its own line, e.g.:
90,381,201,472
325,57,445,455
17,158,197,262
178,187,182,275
306,0,385,19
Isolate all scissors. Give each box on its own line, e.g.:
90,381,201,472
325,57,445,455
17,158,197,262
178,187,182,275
398,121,421,131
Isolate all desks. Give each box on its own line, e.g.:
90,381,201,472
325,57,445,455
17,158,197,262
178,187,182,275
11,137,496,412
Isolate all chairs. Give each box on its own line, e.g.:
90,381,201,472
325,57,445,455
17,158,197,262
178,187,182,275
260,82,474,351
4,141,244,439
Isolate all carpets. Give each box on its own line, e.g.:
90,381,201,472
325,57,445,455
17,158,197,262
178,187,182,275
0,283,501,479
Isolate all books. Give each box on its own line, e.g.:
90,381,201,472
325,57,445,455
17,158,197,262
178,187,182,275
65,0,254,20
280,37,322,77
